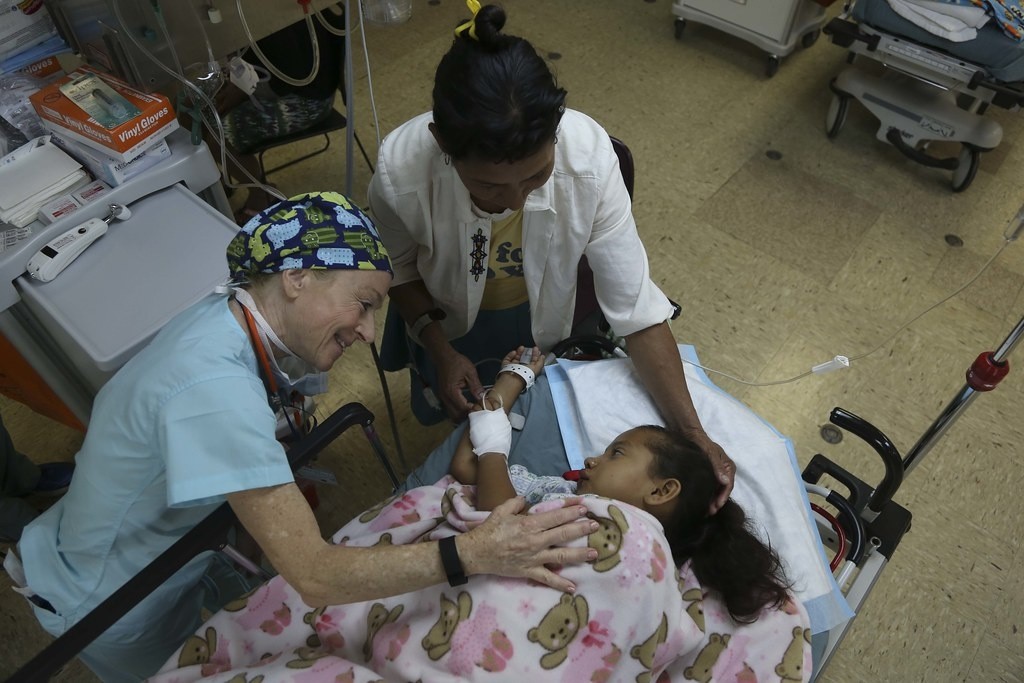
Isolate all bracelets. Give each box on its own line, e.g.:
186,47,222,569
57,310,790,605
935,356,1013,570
405,314,434,348
496,363,535,391
438,534,468,587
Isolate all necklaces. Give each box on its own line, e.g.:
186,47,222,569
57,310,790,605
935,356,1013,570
239,302,310,439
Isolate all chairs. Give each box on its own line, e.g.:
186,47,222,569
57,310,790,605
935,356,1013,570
215,2,374,209
378,134,634,376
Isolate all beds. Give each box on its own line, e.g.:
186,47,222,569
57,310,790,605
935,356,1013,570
823,0,1024,193
0,332,911,683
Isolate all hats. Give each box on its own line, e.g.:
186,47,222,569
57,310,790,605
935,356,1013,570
224,189,395,284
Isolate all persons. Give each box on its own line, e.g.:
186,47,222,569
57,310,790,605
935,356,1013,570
3,191,599,683
0,414,76,541
367,0,737,516
147,346,790,683
